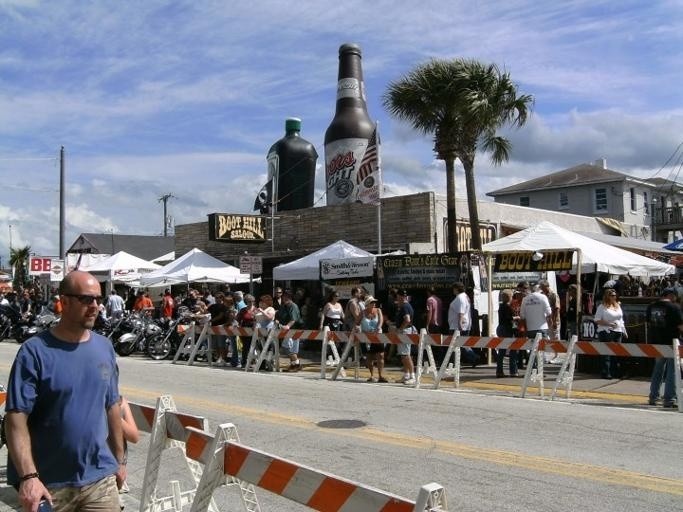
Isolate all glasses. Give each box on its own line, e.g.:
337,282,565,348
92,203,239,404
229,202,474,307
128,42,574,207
60,293,104,305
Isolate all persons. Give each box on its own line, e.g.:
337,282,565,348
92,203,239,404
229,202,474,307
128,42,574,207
100,288,320,372
495,277,683,408
0,287,61,320
316,281,472,386
3,270,128,512
106,364,138,464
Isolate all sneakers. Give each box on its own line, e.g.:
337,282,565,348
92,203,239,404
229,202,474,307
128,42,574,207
646,402,678,409
366,377,388,384
496,373,507,378
394,376,415,385
288,361,302,373
510,373,525,378
213,356,274,372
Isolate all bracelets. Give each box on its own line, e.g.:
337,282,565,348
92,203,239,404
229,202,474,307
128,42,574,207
115,457,127,465
17,473,40,481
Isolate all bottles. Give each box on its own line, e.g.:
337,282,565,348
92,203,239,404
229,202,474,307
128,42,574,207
266,117,317,211
323,42,376,207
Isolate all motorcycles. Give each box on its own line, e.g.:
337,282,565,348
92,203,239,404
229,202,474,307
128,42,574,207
0,292,220,365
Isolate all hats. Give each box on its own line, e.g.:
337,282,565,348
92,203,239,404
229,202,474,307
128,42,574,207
364,296,378,306
